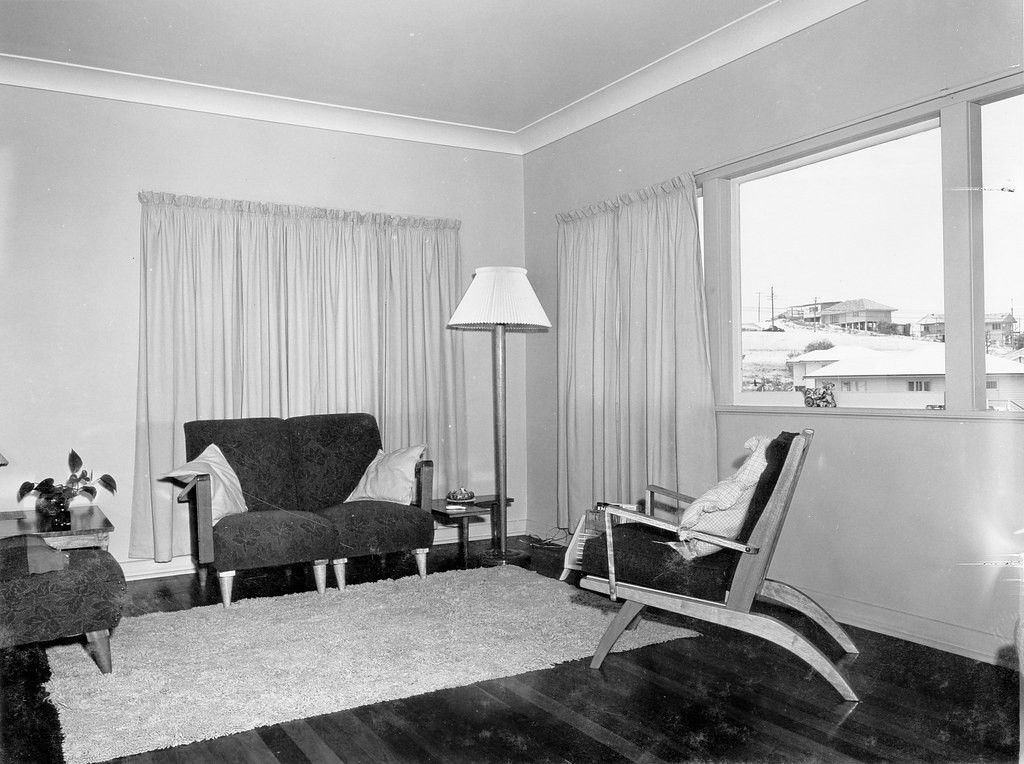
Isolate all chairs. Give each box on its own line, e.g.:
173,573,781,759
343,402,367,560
580,428,861,703
159,412,436,609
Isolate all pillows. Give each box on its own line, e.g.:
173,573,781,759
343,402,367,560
659,433,777,562
342,445,427,508
165,442,249,529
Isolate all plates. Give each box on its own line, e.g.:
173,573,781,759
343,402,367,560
447,497,477,503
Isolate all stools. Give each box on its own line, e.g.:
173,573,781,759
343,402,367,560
0,534,127,674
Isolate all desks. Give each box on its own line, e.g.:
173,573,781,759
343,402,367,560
0,505,115,552
430,494,515,561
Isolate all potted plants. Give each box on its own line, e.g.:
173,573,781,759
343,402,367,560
13,449,117,519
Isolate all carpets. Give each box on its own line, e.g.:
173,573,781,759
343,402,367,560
39,563,708,764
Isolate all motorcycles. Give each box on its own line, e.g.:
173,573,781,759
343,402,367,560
803,381,836,408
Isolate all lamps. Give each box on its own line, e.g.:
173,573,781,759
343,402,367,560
447,266,551,568
